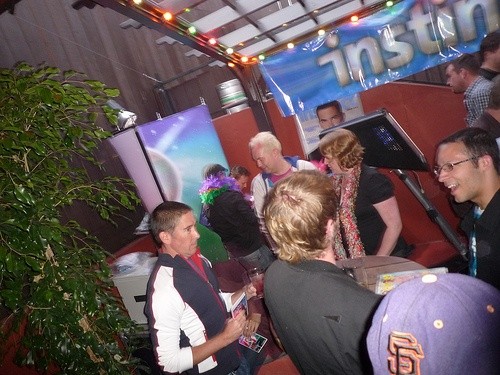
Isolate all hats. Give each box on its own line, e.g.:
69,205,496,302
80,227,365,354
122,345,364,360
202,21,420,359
204,164,228,179
366,273,500,375
487,75,500,109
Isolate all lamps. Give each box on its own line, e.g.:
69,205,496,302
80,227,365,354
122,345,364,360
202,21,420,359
102,99,137,130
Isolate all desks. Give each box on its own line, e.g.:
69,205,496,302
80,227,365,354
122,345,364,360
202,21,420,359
336,256,428,293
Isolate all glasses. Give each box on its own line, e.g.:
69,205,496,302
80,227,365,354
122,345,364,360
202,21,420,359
432,155,486,176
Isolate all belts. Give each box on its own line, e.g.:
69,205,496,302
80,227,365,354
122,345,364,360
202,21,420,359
226,364,240,375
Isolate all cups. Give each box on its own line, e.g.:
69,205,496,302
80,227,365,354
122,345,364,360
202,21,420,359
343,258,371,288
242,267,268,299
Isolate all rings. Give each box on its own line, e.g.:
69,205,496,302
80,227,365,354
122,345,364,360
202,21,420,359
246,326,249,330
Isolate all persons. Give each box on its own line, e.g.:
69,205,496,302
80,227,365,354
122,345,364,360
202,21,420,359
249,132,319,257
471,75,500,139
316,100,345,130
195,222,279,364
262,169,386,375
445,53,493,127
198,163,276,275
144,200,257,375
199,166,249,231
476,31,500,80
427,127,500,290
317,129,417,259
